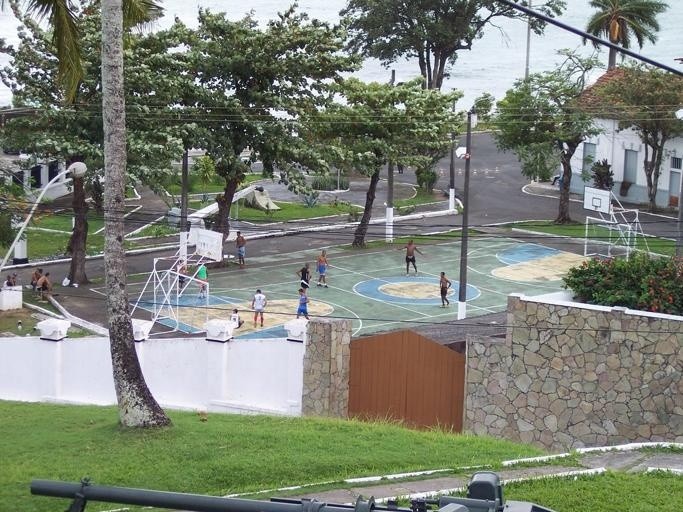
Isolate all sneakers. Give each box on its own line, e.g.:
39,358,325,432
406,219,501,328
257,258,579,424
317,284,328,288
440,301,449,307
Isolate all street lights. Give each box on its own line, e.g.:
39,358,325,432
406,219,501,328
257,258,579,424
453,105,481,321
674,107,683,259
0,159,89,274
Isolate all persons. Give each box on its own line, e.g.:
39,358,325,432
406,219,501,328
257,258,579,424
229,308,244,330
195,260,209,298
176,258,186,296
295,263,312,295
2,268,57,297
251,289,267,328
397,164,404,174
439,272,451,308
296,289,310,320
315,250,329,288
235,231,246,267
396,239,426,277
